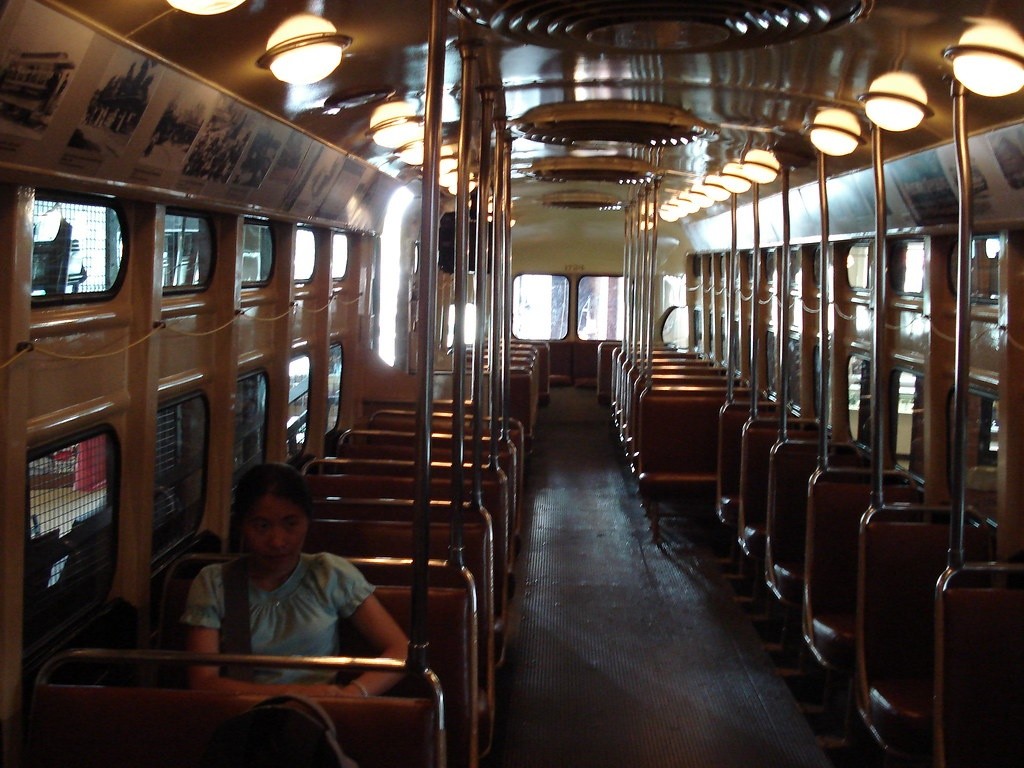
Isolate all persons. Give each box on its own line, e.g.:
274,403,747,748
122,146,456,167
182,462,412,700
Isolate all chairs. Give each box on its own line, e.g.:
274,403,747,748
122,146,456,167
26,342,1024,768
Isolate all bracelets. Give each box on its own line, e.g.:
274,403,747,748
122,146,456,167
350,680,369,699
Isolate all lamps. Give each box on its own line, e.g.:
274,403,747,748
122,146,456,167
659,24,1023,221
254,15,493,224
